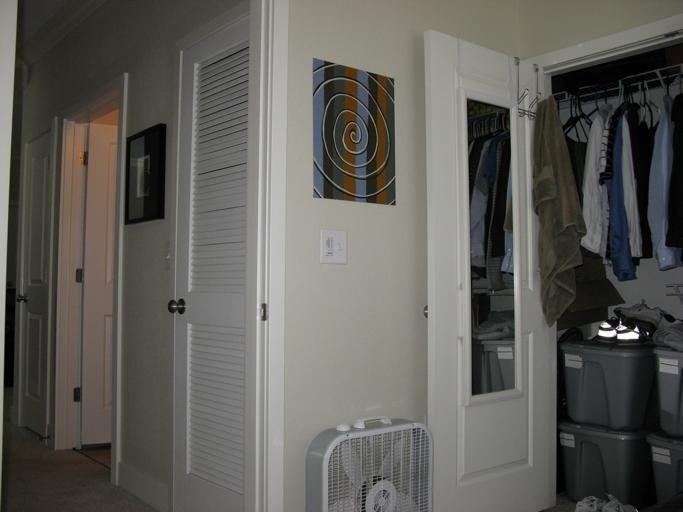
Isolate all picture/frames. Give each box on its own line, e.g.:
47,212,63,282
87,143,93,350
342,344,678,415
125,123,165,225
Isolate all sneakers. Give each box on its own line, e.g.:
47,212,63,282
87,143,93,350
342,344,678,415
574,494,638,512
597,300,683,352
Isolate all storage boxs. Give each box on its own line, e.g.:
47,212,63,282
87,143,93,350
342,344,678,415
556,341,682,508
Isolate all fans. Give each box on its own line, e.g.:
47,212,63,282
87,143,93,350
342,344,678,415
302,417,433,512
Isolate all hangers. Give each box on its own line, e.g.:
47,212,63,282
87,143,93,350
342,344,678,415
547,70,683,149
468,107,510,162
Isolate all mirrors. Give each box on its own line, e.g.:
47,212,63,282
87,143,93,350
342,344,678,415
456,86,521,399
